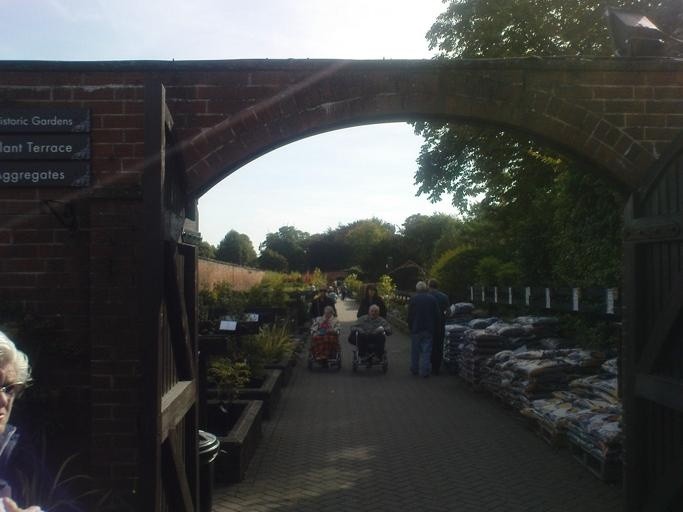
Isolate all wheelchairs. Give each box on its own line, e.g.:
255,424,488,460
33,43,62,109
307,329,342,370
352,331,388,373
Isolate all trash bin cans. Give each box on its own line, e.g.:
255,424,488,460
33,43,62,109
198,430,228,512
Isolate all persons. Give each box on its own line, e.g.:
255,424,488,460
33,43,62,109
0,329,82,512
308,279,394,371
426,279,451,376
406,281,442,377
